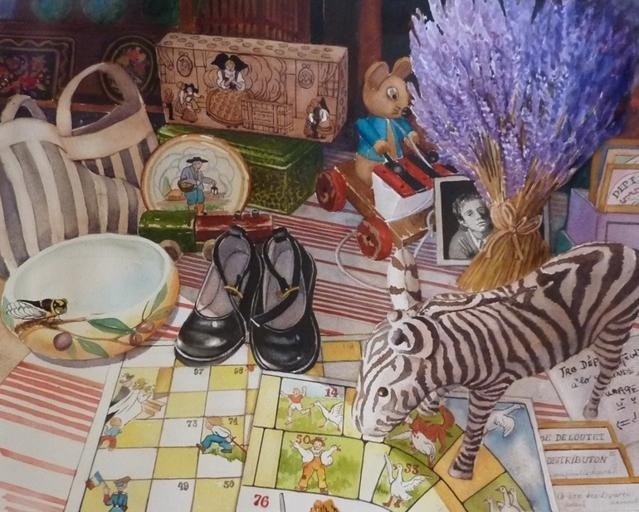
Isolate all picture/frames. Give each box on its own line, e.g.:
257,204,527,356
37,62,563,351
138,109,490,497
432,176,549,267
588,140,639,215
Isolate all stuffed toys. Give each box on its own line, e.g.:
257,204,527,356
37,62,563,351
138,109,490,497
349,240,639,481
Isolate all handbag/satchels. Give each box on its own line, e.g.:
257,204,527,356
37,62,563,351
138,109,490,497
0,62,159,281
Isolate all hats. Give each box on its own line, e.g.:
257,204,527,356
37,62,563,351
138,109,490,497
187,157,208,163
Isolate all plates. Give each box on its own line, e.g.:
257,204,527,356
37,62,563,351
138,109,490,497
139,134,252,219
3,233,181,363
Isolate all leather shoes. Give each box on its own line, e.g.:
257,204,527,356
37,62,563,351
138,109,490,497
249,227,320,373
174,225,262,368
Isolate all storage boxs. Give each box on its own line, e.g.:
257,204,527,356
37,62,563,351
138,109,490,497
153,32,347,213
565,190,637,253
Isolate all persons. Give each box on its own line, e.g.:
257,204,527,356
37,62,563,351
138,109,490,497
450,193,494,260
